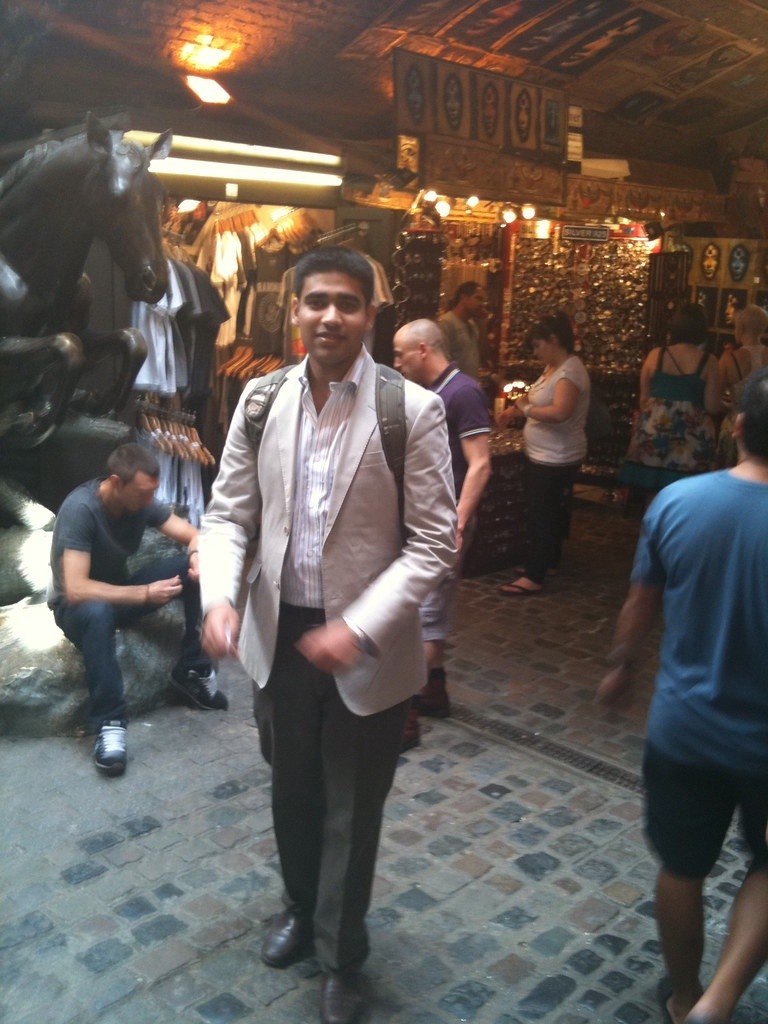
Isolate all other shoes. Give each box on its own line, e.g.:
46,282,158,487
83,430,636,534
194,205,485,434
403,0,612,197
401,709,420,752
410,669,450,717
658,971,703,1024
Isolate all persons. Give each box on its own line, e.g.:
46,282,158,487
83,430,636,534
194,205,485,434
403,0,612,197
388,319,493,723
678,304,721,356
436,283,496,386
710,305,768,472
616,315,721,521
51,445,231,776
592,364,767,1024
190,246,457,1024
497,310,590,599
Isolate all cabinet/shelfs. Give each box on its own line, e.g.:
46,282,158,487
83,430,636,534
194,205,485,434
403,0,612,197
408,218,768,576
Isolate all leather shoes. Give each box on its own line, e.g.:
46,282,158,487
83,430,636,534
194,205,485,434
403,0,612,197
261,909,313,967
319,970,366,1024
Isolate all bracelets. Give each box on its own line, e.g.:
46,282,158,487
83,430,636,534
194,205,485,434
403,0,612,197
521,403,533,416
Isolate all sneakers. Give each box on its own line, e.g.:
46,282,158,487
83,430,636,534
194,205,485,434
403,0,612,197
168,662,229,711
94,719,128,770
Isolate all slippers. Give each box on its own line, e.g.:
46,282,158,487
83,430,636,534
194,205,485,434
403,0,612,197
498,579,544,596
513,568,556,577
